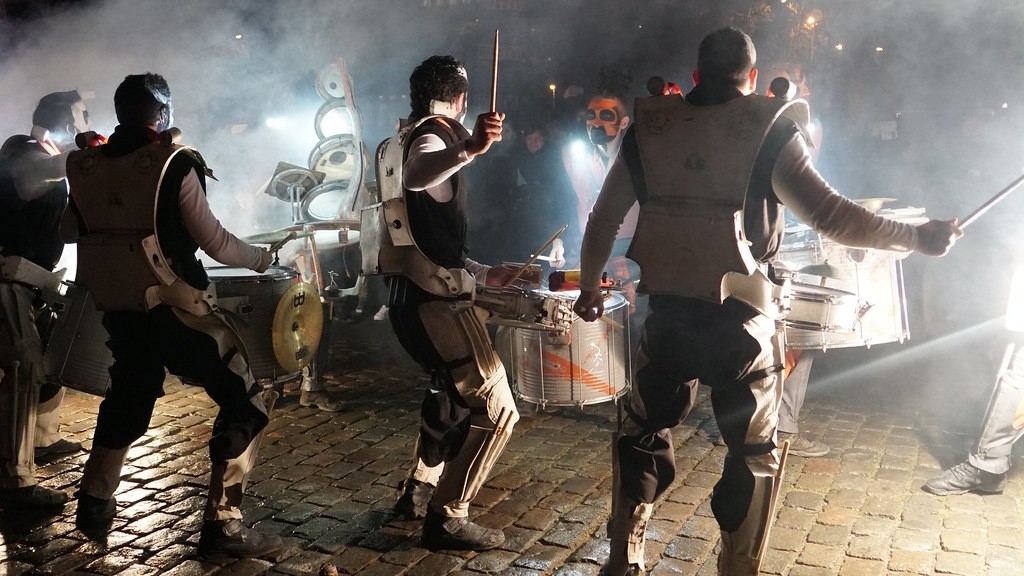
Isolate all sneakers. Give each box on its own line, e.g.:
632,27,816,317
716,435,726,447
776,431,830,457
924,459,1007,495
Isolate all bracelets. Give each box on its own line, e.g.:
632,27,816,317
580,284,600,291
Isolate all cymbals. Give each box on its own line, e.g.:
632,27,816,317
485,315,563,332
271,281,323,373
237,228,316,245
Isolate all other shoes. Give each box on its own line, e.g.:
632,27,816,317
395,479,433,518
35,438,81,458
73,489,117,525
2,484,70,509
196,521,284,560
417,512,505,550
299,389,344,412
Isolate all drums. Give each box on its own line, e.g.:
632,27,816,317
474,284,575,333
176,264,302,389
34,279,119,397
502,262,632,407
770,204,931,351
296,230,363,291
264,60,364,222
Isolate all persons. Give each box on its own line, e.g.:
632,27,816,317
299,354,344,412
923,255,1022,498
58,72,282,558
481,21,966,575
0,91,94,516
373,53,519,551
